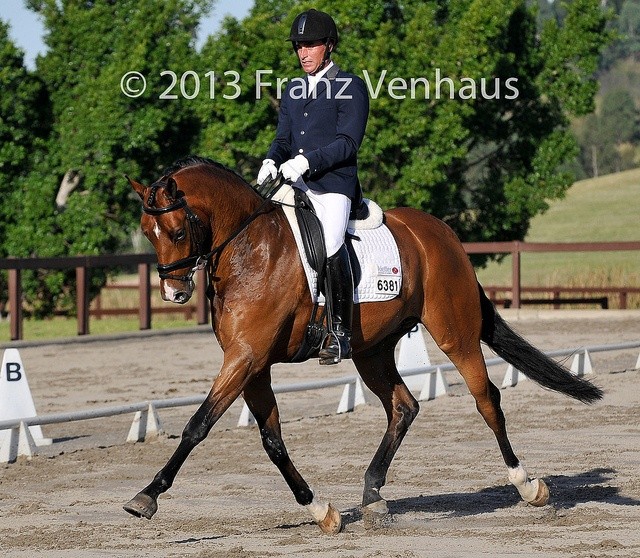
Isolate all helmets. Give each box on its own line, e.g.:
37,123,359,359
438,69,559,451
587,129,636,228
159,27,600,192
285,8,339,42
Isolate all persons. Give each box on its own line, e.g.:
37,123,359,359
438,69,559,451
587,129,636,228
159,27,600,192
256,8,369,361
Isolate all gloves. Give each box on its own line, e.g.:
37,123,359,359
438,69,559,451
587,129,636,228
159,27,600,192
257,158,278,185
278,154,309,182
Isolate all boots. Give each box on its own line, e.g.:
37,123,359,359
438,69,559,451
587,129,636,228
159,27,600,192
319,242,354,359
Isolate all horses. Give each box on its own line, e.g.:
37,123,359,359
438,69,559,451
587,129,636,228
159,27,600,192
123,157,604,541
